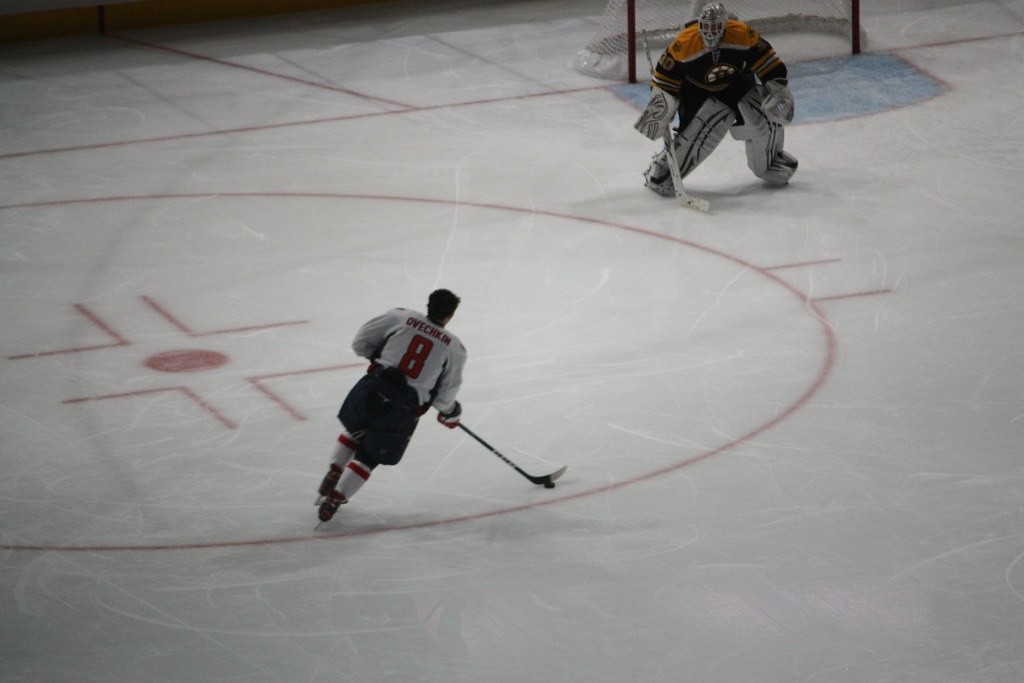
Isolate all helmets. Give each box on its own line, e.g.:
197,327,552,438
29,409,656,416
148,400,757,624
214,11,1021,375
698,2,727,48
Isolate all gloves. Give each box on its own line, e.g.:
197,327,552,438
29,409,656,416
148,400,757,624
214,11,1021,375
635,86,679,141
437,400,463,430
761,78,795,126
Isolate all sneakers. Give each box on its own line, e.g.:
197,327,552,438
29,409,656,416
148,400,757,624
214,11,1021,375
314,490,348,532
316,470,342,507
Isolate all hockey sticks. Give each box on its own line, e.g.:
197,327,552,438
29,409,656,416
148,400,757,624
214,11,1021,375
457,422,567,485
640,27,710,211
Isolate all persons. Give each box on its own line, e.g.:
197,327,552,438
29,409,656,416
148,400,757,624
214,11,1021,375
635,0,798,198
315,289,468,528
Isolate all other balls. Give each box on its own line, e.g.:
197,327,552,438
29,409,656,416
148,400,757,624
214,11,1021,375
544,481,555,488
